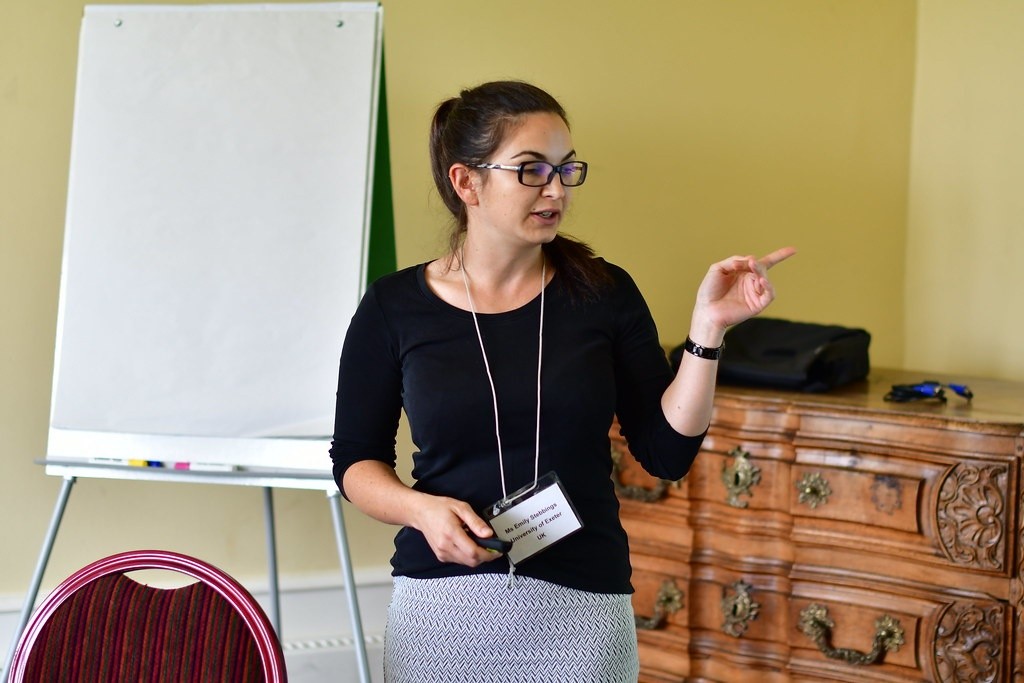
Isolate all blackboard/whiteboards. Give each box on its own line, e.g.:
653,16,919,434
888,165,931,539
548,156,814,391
44,1,385,492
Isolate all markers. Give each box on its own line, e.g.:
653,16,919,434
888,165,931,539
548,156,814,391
468,534,514,555
174,462,239,474
87,457,164,468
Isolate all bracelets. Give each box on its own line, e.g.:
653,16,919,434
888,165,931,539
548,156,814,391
685,335,725,360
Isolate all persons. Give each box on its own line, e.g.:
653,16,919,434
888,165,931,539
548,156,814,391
329,81,795,683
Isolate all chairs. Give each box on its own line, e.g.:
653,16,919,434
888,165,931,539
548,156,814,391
5,550,288,683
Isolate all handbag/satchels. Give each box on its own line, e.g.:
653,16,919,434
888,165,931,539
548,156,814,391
669,318,872,398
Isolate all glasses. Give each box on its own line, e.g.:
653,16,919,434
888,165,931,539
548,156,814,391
462,161,588,188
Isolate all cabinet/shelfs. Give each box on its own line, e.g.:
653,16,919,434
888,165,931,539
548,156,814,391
606,367,1024,683
